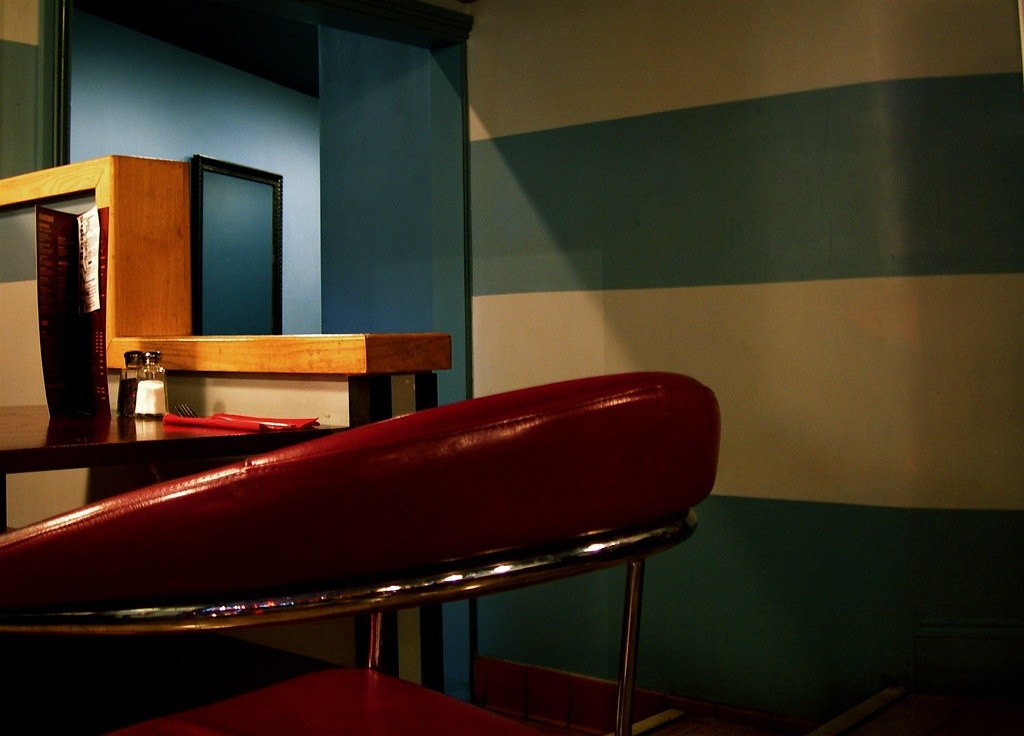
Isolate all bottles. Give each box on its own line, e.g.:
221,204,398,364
116,350,169,420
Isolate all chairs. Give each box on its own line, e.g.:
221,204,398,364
0,372,723,736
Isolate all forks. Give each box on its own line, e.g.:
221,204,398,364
174,403,296,427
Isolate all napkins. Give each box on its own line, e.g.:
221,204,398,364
164,412,321,432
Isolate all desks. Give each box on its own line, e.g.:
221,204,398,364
0,403,349,533
